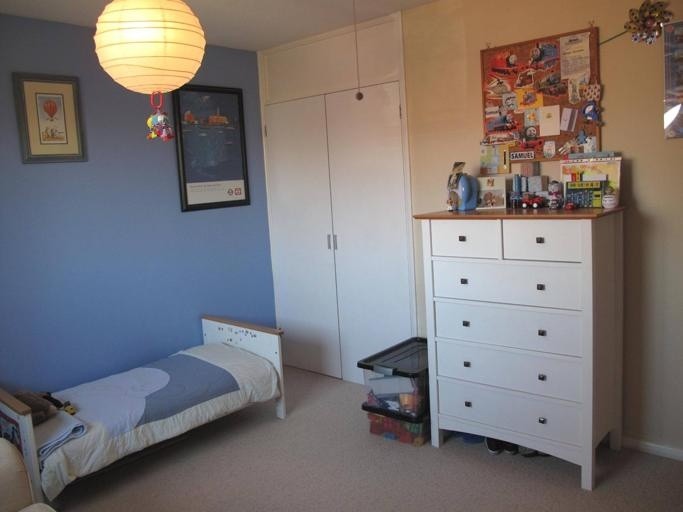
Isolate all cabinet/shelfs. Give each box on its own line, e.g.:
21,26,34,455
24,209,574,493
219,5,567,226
413,207,627,490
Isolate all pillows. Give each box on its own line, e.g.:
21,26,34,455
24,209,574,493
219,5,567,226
13,391,56,427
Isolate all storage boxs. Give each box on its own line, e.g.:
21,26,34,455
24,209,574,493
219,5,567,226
357,336,428,446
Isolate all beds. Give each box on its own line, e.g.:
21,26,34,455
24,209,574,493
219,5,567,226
0,315,286,503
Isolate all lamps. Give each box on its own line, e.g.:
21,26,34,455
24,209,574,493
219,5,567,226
93,0,206,94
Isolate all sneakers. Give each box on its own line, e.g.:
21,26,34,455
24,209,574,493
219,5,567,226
453,432,551,457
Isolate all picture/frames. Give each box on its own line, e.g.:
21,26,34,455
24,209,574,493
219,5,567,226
171,83,251,212
12,72,87,163
